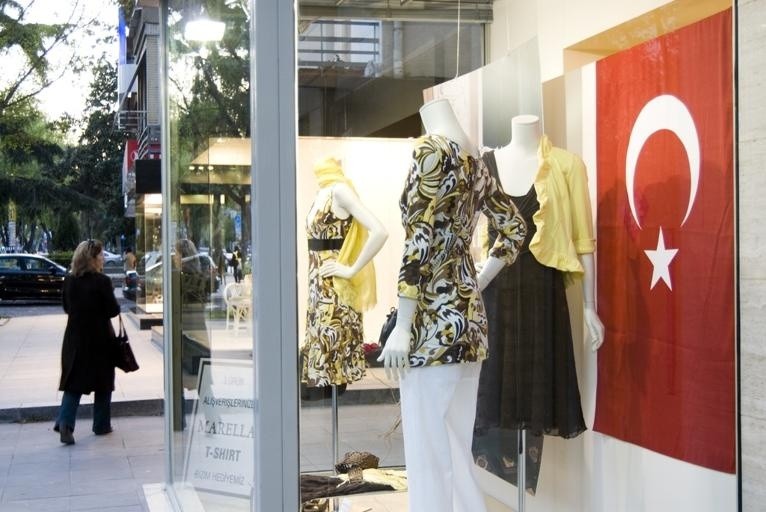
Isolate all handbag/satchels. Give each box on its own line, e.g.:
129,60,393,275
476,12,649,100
228,260,237,265
116,334,139,373
379,307,398,351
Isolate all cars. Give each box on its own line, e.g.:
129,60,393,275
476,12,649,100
102,250,122,266
122,260,222,302
0,253,70,302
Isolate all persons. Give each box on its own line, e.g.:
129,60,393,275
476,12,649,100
53,240,121,445
376,97,529,512
120,247,137,274
301,153,388,389
219,250,229,287
481,114,604,439
228,245,243,283
174,239,222,436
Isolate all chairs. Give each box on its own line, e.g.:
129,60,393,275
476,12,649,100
224,282,250,333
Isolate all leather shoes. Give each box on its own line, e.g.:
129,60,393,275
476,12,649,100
59,425,74,444
95,427,114,436
